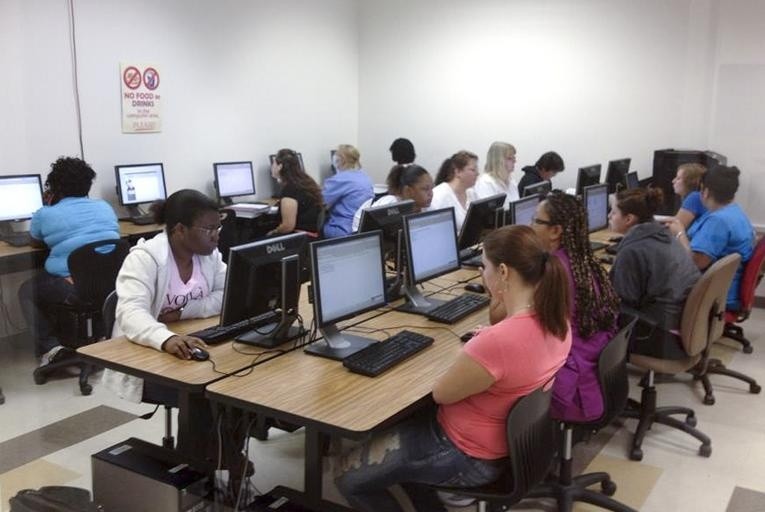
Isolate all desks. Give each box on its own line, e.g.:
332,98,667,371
1,198,281,404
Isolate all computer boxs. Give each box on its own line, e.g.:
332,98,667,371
91,437,214,512
653,147,726,195
240,485,362,512
50,309,104,376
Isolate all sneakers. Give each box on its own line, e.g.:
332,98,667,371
33,344,69,374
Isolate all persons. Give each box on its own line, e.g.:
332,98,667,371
531,195,623,423
421,149,479,238
607,185,703,359
475,141,521,211
256,149,323,241
20,157,120,384
318,144,376,238
389,139,416,163
332,225,573,510
112,189,257,476
518,151,564,197
672,163,708,231
352,165,434,234
668,164,756,312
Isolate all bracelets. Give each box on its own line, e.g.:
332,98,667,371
270,226,282,234
675,230,684,239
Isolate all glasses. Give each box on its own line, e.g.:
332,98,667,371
180,224,222,235
531,214,556,228
506,155,516,161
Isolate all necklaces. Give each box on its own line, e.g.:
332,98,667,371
504,300,534,317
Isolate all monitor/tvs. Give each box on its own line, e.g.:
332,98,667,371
269,152,306,176
213,161,257,207
509,193,543,228
358,199,416,262
623,171,642,189
0,174,47,235
458,192,506,249
585,183,608,234
399,206,461,313
217,232,309,346
605,158,630,194
303,229,388,358
576,164,601,194
522,180,551,199
115,163,170,223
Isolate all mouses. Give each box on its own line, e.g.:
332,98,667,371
189,346,209,361
465,283,484,293
598,255,614,264
460,331,475,341
610,235,623,242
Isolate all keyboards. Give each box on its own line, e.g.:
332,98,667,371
188,313,289,343
133,216,157,226
343,330,434,378
5,236,39,246
426,292,491,324
